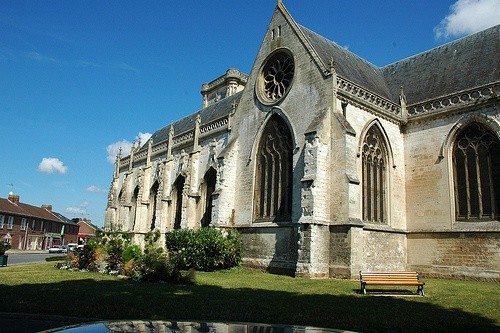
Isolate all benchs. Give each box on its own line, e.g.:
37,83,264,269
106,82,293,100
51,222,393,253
358,270,425,297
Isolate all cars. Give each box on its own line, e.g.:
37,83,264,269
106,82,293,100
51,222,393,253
48,242,84,253
